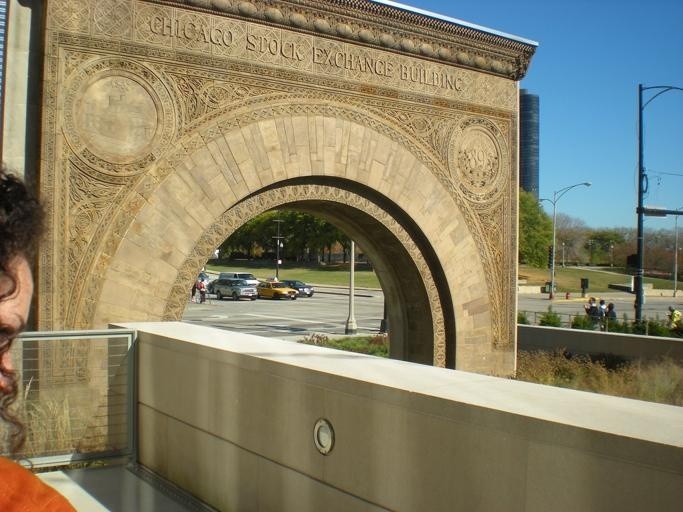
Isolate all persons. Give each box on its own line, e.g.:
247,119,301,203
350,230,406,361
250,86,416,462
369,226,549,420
584,297,598,326
0,170,76,511
598,299,606,329
604,303,616,322
668,304,682,329
191,266,209,304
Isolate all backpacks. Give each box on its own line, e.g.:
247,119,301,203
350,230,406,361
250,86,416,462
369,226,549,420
197,282,204,290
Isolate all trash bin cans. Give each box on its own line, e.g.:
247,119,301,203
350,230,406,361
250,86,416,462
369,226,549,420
541,282,557,293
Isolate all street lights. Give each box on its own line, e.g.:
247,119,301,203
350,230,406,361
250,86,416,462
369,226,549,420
540,183,591,299
562,242,565,268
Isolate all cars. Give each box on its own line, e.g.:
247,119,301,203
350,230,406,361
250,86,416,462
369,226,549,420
198,272,314,300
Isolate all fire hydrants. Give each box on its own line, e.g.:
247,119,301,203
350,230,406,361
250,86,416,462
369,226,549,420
566,291,570,299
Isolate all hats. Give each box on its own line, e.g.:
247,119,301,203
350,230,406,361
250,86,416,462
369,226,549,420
669,305,675,311
